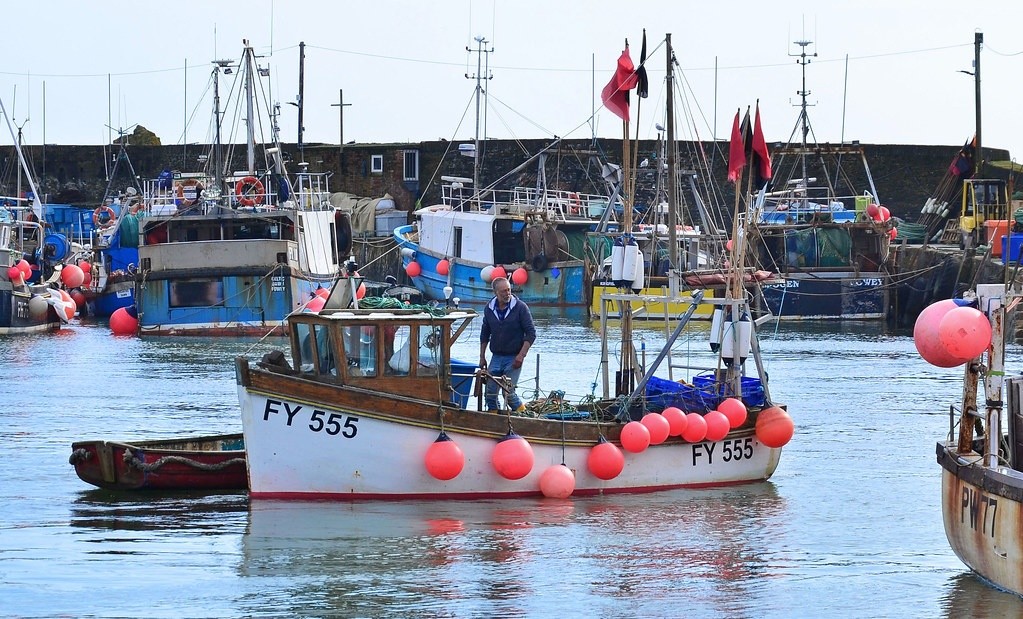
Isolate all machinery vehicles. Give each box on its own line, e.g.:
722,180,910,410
958,177,1012,251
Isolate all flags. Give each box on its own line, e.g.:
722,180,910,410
600,34,649,121
947,137,975,178
726,108,772,185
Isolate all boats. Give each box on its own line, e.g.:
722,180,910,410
70,434,250,494
934,171,1022,597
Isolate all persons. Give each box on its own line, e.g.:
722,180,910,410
479,277,536,414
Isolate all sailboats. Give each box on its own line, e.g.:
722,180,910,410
0,0,894,507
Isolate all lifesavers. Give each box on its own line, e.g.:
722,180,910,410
235,177,265,207
93,206,115,229
567,194,579,212
133,205,144,216
177,180,203,206
